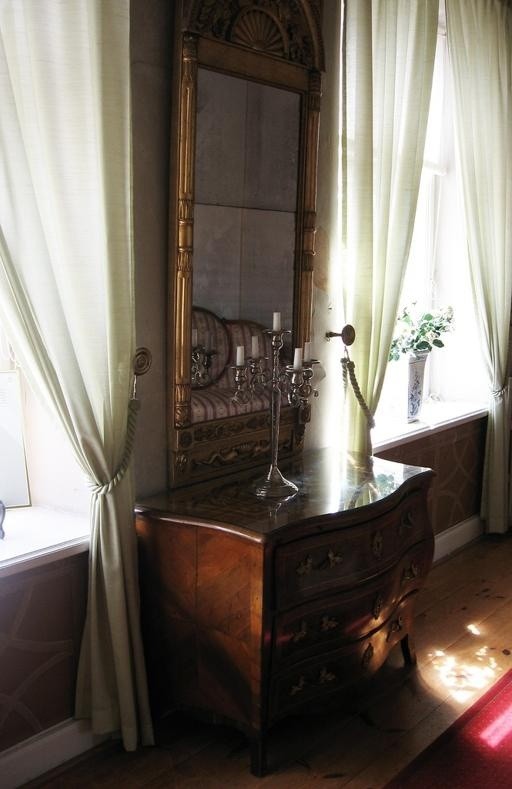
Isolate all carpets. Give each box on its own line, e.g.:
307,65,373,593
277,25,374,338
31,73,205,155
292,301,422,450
381,671,511,789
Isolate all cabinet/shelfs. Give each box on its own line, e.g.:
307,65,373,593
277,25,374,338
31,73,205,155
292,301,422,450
129,446,436,777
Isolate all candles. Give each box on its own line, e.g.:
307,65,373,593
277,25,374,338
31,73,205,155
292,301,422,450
191,327,213,352
270,311,282,332
292,341,313,371
234,335,262,367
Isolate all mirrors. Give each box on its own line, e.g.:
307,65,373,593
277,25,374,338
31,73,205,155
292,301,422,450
163,1,324,491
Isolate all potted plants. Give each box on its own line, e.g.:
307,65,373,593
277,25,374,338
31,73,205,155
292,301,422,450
380,300,458,426
